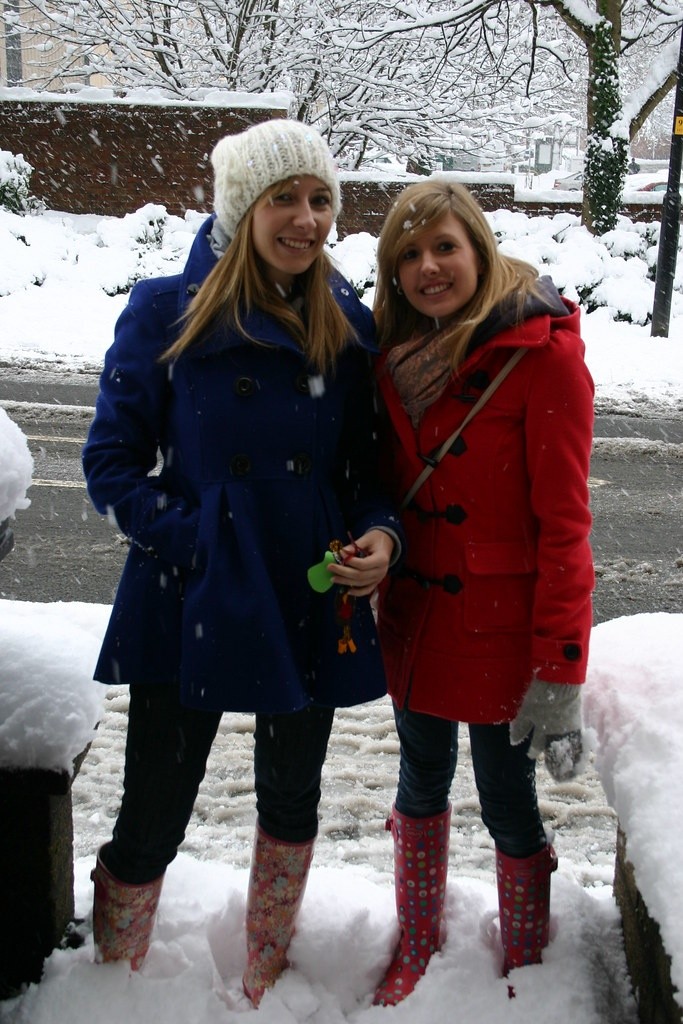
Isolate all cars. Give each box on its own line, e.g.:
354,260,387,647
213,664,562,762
553,171,583,191
636,182,668,191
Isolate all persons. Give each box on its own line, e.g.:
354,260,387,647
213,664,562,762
80,119,409,1008
374,180,595,1007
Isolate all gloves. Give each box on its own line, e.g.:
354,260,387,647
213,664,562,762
510,678,587,783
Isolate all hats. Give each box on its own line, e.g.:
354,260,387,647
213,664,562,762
210,119,343,240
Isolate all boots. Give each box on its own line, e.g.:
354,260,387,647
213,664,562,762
374,802,452,1007
241,817,319,1012
495,843,561,998
89,843,168,973
